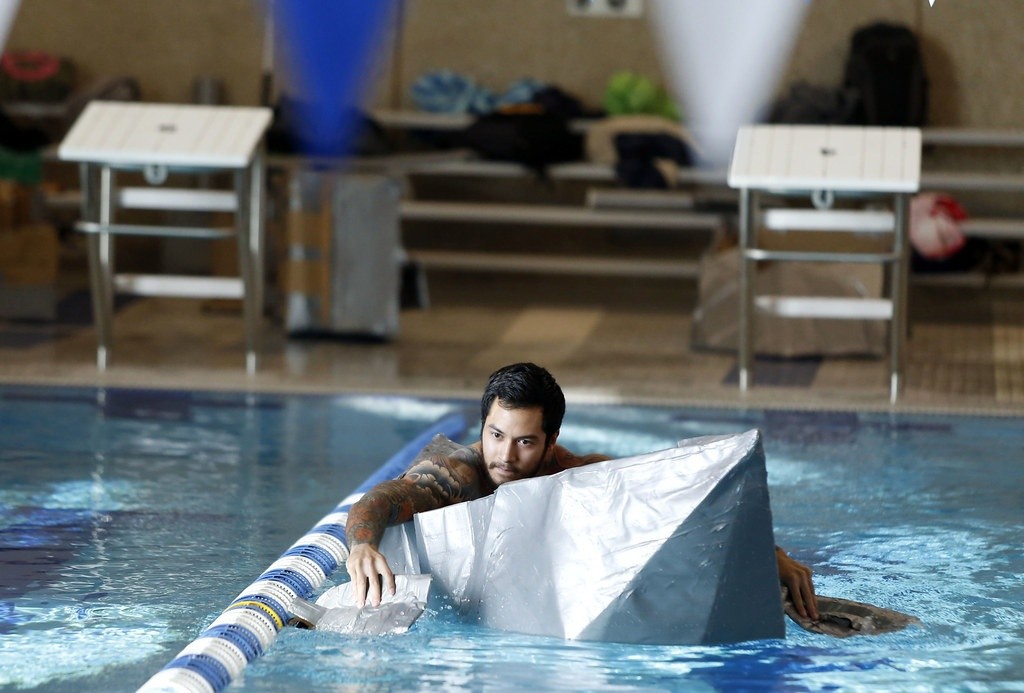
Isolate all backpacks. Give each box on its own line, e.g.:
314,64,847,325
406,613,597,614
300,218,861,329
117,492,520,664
839,19,929,130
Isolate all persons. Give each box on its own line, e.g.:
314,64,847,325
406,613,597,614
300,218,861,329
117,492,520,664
346,362,819,620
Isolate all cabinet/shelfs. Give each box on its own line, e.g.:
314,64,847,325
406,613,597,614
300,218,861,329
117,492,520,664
372,109,1023,275
